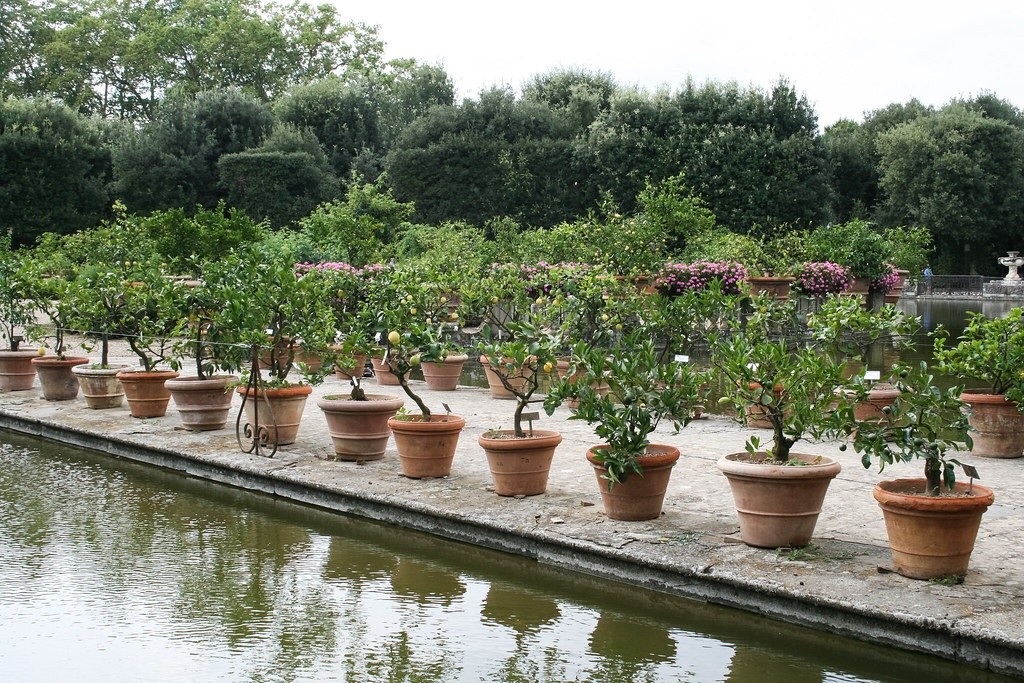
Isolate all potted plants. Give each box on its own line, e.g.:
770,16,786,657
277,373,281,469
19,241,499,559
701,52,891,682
2,209,1024,586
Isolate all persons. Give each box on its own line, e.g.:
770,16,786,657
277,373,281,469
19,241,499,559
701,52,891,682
924,263,933,294
